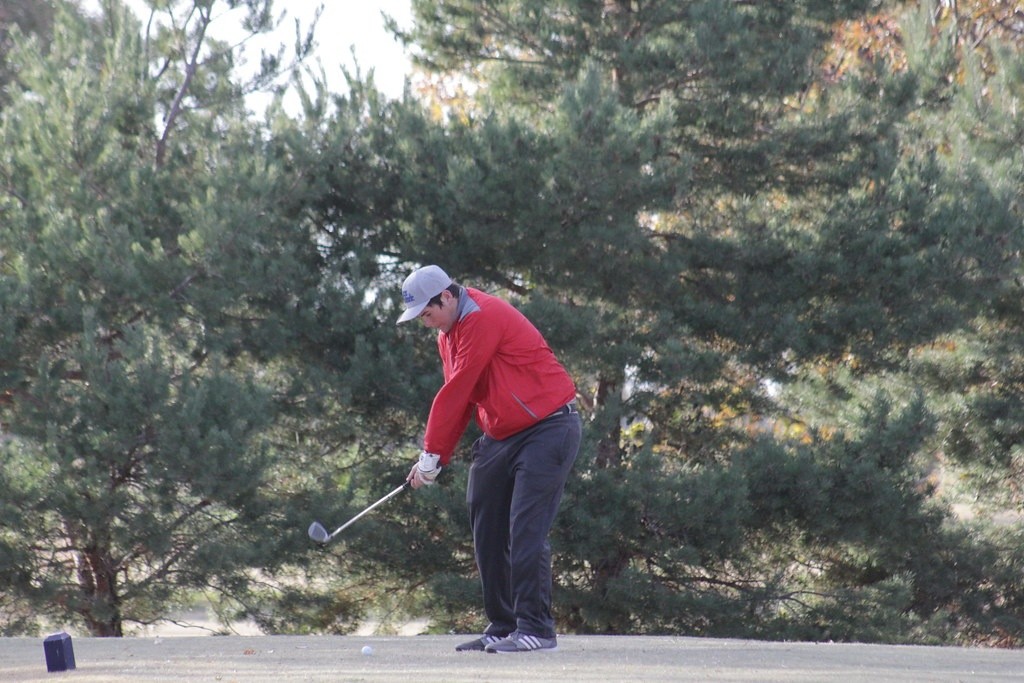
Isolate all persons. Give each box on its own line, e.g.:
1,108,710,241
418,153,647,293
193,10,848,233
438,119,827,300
395,264,583,655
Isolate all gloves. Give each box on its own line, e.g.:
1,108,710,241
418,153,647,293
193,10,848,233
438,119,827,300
418,450,443,485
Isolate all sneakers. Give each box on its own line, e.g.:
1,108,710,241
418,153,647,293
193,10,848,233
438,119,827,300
484,629,558,654
455,634,506,652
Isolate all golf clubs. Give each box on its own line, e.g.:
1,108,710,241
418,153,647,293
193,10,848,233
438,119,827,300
308,480,410,545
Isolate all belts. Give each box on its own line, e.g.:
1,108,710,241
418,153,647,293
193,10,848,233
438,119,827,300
544,403,577,420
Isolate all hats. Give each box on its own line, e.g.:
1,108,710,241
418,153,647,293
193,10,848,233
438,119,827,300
396,264,453,326
